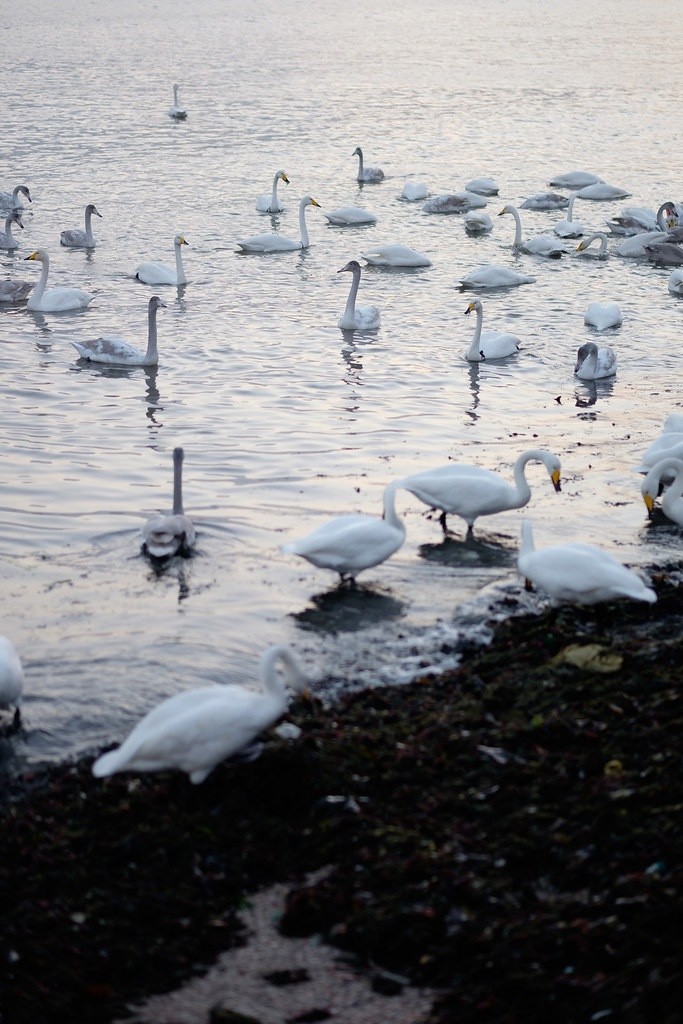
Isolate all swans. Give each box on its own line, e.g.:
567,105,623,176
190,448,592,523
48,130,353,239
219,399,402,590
584,301,622,331
276,480,406,583
141,447,196,566
1,630,25,730
0,148,683,313
464,298,523,362
630,414,683,528
169,84,187,117
337,260,380,330
68,296,168,365
517,520,657,606
402,450,561,536
92,643,315,785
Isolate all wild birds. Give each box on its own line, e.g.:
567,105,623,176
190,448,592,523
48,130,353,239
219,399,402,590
573,342,616,380
